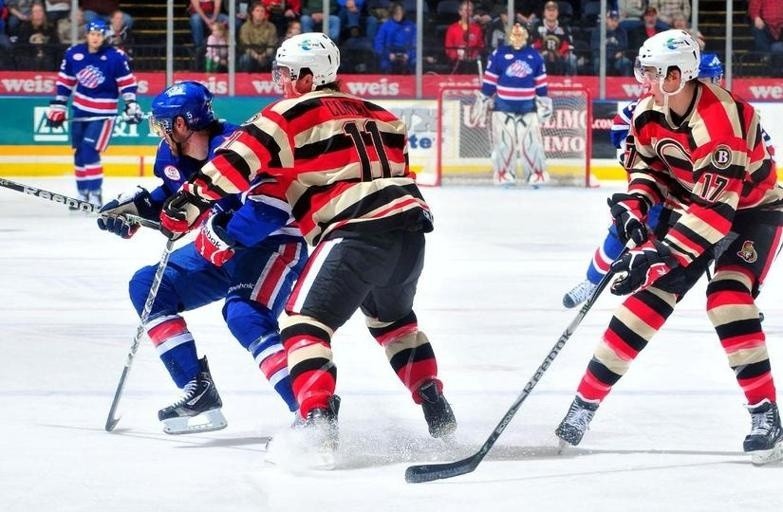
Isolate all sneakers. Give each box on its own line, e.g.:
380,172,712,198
564,280,596,308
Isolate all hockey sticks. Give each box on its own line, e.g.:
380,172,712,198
101,230,177,431
405,238,640,484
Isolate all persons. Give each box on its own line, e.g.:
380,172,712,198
555,26,783,449
98,80,311,422
163,30,458,455
187,1,439,74
747,1,783,75
439,0,724,74
561,52,724,309
1,1,134,71
469,21,554,189
45,18,144,209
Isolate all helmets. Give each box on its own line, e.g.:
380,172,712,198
87,17,111,30
699,53,723,79
634,30,701,97
273,32,341,93
151,82,216,130
510,24,529,50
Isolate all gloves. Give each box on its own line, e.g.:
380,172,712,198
472,90,491,128
46,108,65,127
607,191,679,295
97,186,236,268
535,96,553,122
123,102,143,124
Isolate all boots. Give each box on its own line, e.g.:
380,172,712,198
419,381,457,439
69,189,102,210
555,393,600,445
158,356,222,420
744,398,783,450
265,395,341,453
